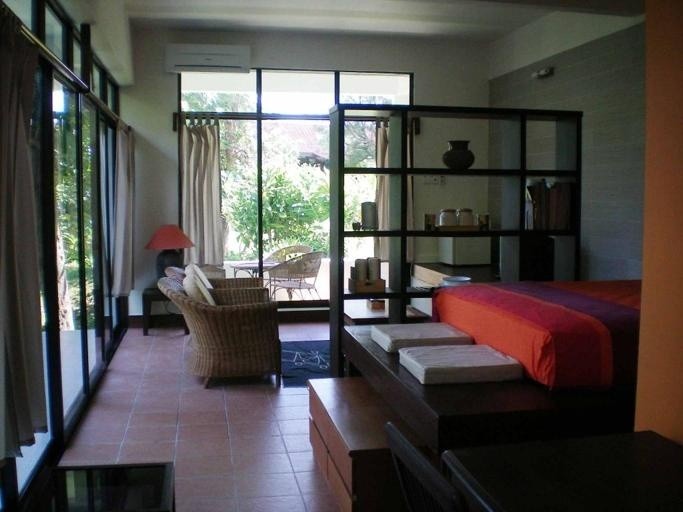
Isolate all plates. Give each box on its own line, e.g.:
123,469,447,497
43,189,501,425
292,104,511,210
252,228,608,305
442,276,471,285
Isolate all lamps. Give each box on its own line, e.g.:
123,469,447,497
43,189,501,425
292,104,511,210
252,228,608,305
144,225,195,280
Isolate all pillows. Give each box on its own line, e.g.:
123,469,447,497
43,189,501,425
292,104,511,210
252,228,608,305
399,343,522,385
372,323,474,352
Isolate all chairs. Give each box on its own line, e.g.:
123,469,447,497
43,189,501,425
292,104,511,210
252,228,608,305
164,266,263,286
266,245,313,262
157,276,281,390
268,250,323,300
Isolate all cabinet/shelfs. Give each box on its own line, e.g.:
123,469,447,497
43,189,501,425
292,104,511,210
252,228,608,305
307,376,411,512
329,102,581,379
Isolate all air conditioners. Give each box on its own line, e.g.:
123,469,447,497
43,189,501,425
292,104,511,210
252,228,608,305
161,40,252,75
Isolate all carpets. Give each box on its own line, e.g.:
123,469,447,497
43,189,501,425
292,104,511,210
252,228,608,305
280,340,342,388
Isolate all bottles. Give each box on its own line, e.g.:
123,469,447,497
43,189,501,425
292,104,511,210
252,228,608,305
457,208,473,225
440,208,458,225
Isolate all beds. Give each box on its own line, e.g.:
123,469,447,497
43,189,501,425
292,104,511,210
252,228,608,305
434,280,641,452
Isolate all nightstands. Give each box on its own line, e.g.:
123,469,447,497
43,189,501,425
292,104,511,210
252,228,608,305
340,324,553,456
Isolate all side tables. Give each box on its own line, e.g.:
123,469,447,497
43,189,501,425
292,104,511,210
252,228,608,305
142,287,190,336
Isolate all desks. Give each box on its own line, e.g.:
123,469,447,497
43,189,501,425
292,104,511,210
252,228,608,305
442,426,683,511
230,261,279,278
